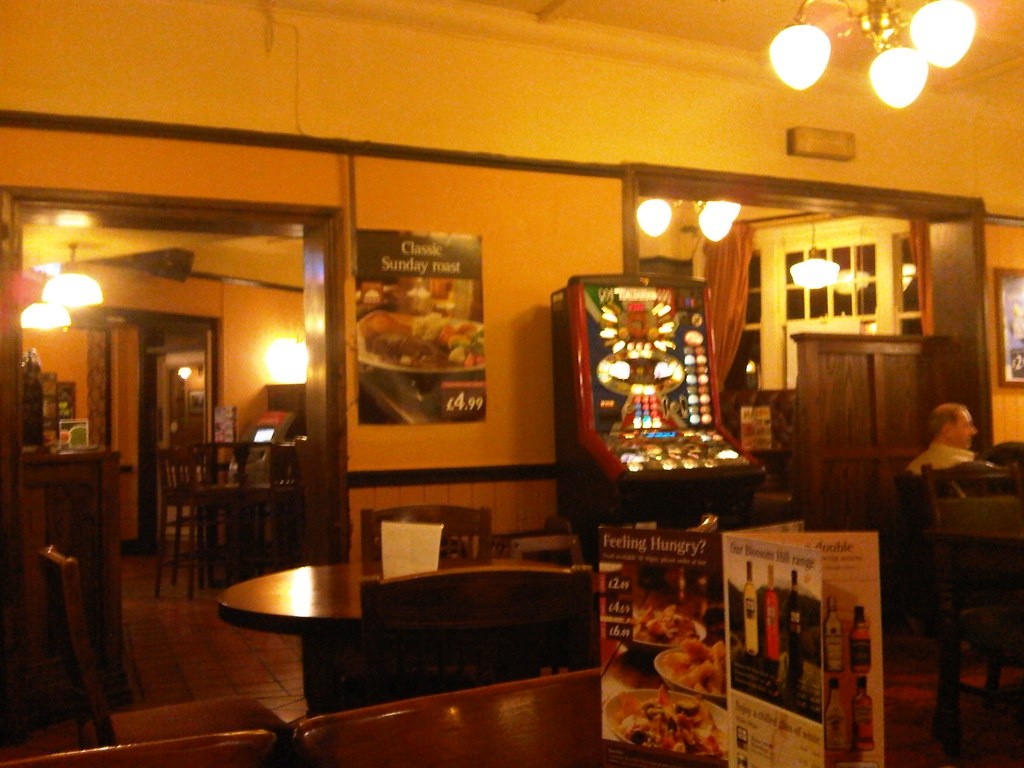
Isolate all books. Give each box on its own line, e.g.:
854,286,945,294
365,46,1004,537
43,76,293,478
599,521,885,768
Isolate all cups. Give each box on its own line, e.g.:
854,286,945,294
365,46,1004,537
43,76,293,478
363,296,380,304
59,418,87,450
405,277,430,314
681,567,708,620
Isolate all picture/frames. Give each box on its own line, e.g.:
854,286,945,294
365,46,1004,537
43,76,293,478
993,266,1024,387
188,390,205,414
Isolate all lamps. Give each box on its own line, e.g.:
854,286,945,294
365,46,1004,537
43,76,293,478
20,243,103,329
636,193,742,240
770,0,978,110
790,214,841,289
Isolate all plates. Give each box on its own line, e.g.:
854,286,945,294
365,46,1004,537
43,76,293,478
355,310,484,372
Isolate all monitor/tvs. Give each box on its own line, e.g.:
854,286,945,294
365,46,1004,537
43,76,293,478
255,427,274,442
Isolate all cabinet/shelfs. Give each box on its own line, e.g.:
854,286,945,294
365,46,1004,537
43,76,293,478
265,384,307,460
785,330,936,538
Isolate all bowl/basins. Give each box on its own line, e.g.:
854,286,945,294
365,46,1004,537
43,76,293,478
602,688,728,755
654,647,728,704
626,614,707,664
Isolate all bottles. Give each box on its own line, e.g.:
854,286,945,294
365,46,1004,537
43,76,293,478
737,753,748,768
784,570,804,677
849,605,871,672
824,597,843,671
764,564,779,660
825,677,847,749
743,560,759,655
852,676,874,750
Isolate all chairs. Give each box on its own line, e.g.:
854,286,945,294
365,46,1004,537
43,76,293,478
898,463,1024,763
0,441,656,768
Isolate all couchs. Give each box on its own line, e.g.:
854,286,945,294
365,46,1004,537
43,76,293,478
720,387,793,477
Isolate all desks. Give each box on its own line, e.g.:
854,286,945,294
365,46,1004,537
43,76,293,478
218,556,587,721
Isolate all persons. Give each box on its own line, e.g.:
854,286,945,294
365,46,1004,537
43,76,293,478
907,403,978,474
